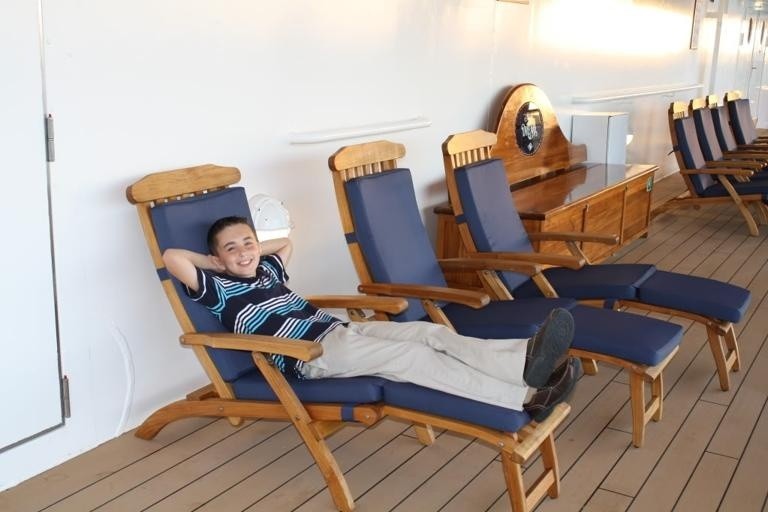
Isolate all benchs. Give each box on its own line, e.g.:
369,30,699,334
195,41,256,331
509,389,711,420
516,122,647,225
434,83,660,293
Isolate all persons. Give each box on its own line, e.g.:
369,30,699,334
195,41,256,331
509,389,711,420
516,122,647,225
160,213,584,425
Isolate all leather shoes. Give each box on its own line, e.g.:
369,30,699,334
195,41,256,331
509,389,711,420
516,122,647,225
521,307,576,389
522,355,582,424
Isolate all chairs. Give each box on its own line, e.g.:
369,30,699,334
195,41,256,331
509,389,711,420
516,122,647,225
123,162,573,512
325,138,685,448
650,91,768,237
440,129,752,394
